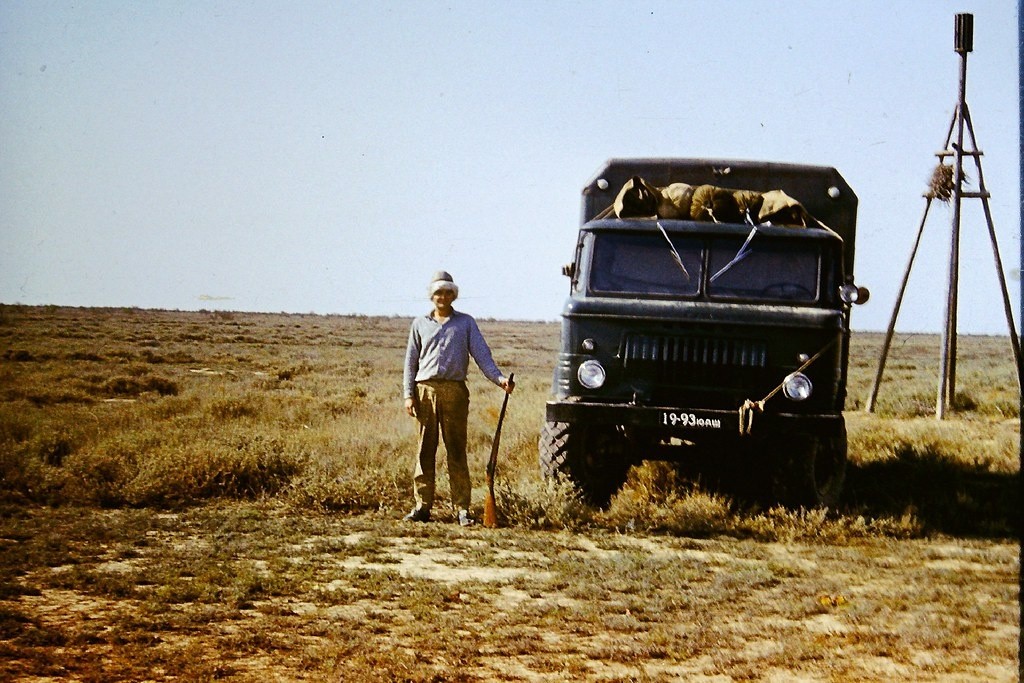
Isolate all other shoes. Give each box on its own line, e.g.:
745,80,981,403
458,510,474,526
403,508,430,522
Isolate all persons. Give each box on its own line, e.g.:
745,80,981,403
403,270,516,526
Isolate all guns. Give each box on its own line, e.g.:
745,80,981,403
481,371,515,528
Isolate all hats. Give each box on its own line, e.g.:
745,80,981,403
429,271,458,301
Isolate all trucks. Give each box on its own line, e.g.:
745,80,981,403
535,155,870,520
